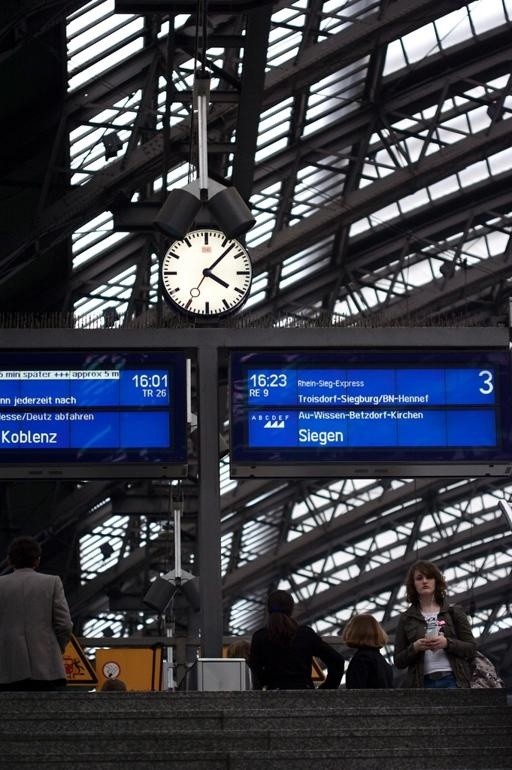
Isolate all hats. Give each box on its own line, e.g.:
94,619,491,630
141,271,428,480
268,590,294,617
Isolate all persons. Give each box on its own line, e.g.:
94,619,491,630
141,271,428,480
242,587,343,690
393,558,478,689
338,613,394,688
1,533,79,687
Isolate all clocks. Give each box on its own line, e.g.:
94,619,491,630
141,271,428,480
162,226,252,319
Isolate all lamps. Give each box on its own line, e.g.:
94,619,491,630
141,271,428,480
142,510,202,613
152,71,256,240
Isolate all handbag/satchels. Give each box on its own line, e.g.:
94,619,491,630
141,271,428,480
454,651,502,689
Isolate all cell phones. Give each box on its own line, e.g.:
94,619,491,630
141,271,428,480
424,628,439,639
